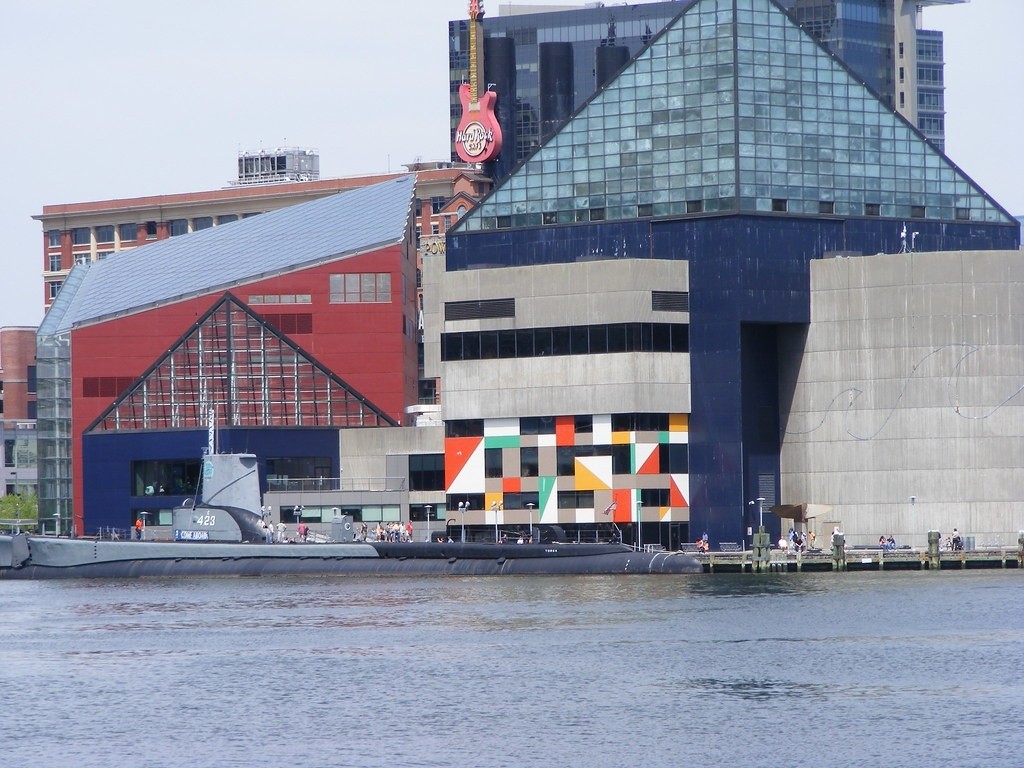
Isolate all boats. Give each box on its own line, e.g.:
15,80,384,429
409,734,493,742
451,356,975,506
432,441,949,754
1,403,704,582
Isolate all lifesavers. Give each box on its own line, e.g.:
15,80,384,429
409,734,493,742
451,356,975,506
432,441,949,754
344,522,351,531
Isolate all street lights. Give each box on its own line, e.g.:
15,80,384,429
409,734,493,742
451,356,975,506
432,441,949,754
53,513,60,537
458,501,470,542
527,502,535,543
140,511,151,540
260,505,270,544
491,501,504,545
10,472,17,495
424,505,433,543
757,497,765,527
635,501,644,551
293,505,305,540
910,496,916,551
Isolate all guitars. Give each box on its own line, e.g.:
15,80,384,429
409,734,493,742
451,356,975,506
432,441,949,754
454,0,504,164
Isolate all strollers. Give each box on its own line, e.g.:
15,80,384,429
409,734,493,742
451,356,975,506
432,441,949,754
958,541,964,551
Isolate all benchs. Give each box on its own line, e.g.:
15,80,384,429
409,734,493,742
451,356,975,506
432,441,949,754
719,542,742,552
644,544,666,552
680,543,701,552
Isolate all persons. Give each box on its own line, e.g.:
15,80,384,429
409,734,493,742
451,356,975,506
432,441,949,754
946,528,962,551
887,535,897,550
516,535,524,545
498,534,507,544
436,535,454,543
377,520,413,541
525,535,532,544
788,528,807,553
297,521,309,544
809,531,815,549
257,519,266,528
276,520,287,544
879,536,888,552
361,522,368,541
778,536,789,552
830,532,834,550
370,527,376,541
135,517,142,540
267,521,275,544
696,532,710,553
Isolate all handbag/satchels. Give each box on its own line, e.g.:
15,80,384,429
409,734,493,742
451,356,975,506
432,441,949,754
385,527,390,532
